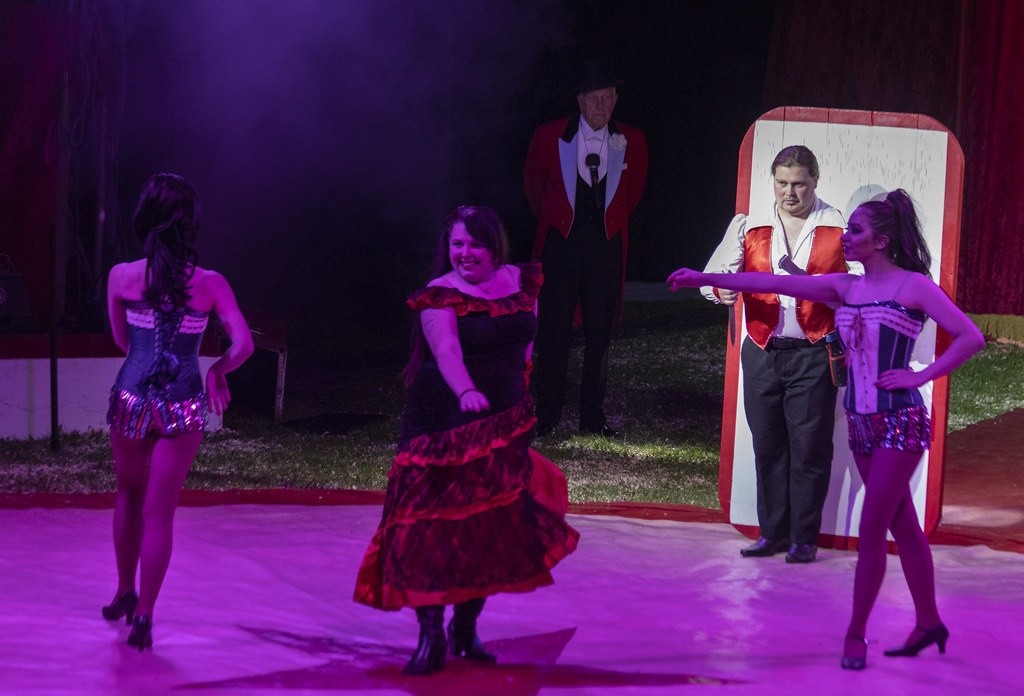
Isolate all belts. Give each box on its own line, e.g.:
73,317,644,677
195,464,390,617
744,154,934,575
768,338,826,350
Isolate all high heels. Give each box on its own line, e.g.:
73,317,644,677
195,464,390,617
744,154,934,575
102,592,138,626
841,636,868,670
128,615,153,652
884,622,950,656
401,606,447,676
447,596,497,661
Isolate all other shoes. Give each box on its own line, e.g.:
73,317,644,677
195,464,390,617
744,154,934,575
579,417,616,436
535,424,557,437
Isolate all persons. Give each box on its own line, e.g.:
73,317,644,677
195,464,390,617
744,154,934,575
667,187,986,669
351,206,581,673
102,174,256,651
698,145,850,562
520,74,648,435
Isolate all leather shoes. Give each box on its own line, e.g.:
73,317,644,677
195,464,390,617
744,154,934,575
740,535,791,557
785,542,818,563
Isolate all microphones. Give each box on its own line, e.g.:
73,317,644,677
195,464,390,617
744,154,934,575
585,153,601,208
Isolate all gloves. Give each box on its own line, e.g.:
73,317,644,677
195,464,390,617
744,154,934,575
717,288,738,305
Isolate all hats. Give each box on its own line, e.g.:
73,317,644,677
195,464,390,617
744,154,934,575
571,61,616,96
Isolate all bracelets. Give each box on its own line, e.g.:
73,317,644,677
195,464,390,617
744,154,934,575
459,389,478,399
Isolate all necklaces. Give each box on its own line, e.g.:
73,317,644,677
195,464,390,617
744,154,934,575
580,123,607,156
478,273,497,294
